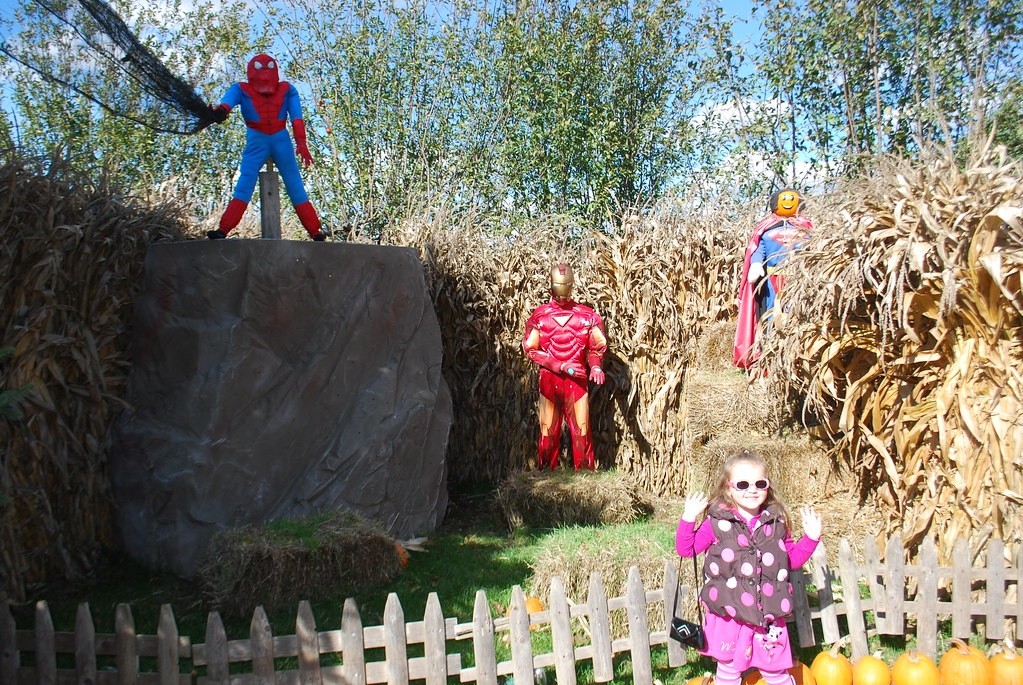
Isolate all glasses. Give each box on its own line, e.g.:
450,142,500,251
725,478,770,490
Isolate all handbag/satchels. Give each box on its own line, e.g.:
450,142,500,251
670,618,704,649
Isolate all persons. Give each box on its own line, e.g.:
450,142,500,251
522,264,607,473
676,449,821,685
207,54,325,241
733,188,813,378
